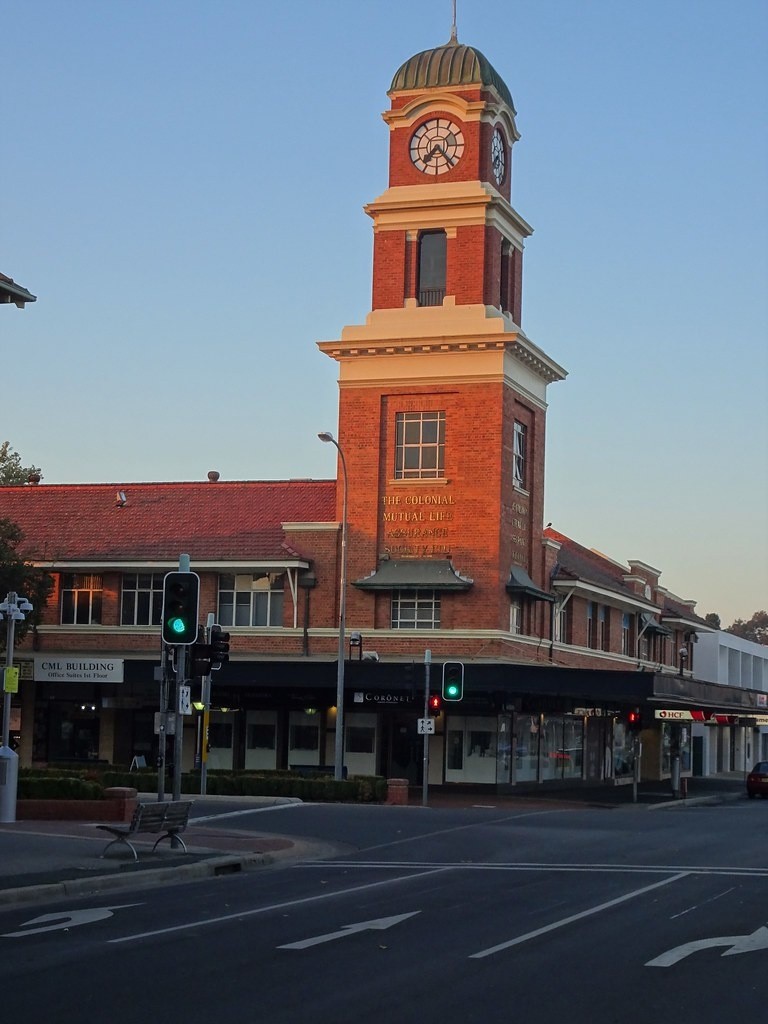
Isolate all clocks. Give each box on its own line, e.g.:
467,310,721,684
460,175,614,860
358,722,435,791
492,128,505,185
409,118,464,175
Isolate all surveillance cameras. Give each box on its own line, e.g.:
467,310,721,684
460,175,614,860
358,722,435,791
11,613,25,624
20,603,33,614
0,613,4,621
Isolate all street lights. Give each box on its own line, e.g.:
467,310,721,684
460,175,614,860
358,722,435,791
194,699,206,772
317,429,347,783
1,591,34,823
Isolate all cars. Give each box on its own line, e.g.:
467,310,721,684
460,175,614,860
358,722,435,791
560,747,628,775
747,761,768,800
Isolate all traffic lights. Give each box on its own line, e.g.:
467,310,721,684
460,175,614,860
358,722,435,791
629,712,637,731
161,571,200,645
211,624,230,672
186,642,213,679
442,661,465,701
431,694,439,707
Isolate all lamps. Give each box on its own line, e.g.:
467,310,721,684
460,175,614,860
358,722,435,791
217,697,232,713
680,648,688,661
117,491,127,506
303,700,320,716
349,631,361,645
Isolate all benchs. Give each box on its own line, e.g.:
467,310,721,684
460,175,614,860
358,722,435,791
96,798,196,863
